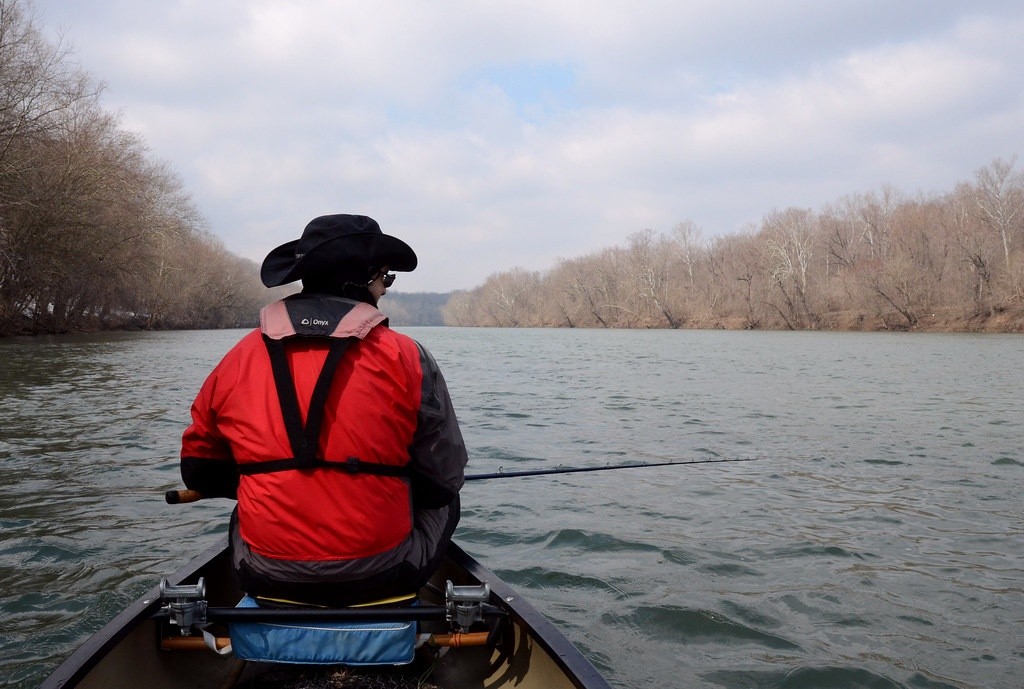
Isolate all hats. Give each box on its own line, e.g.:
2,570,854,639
260,214,418,288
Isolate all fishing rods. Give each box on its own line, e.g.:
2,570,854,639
165,455,758,505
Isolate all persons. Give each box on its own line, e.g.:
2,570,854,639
180,214,469,605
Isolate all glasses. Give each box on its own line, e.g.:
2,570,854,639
378,271,396,288
